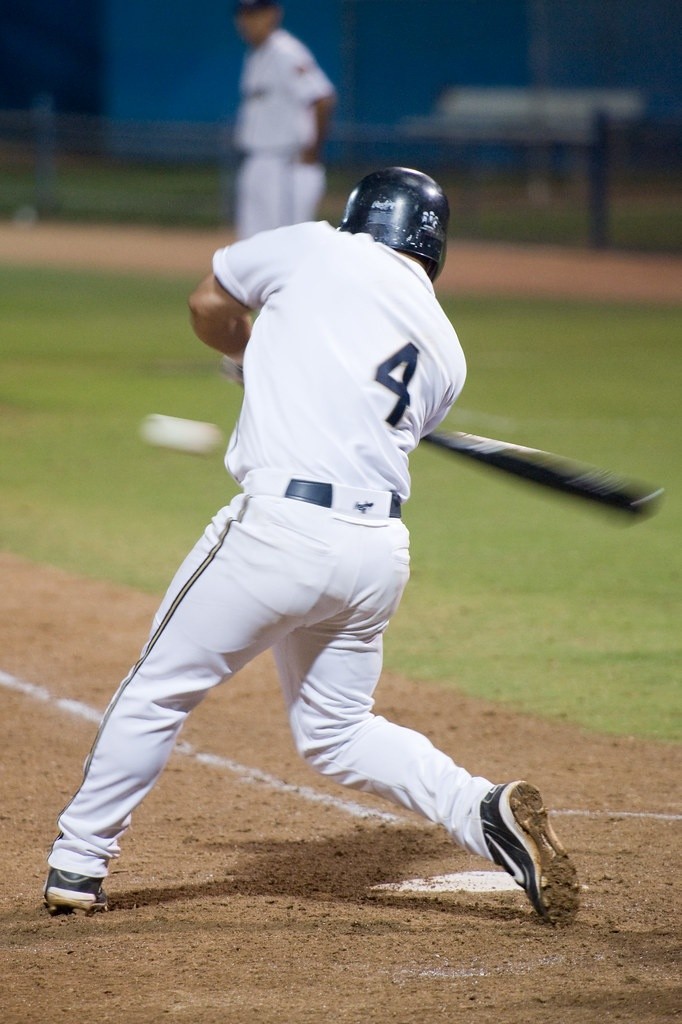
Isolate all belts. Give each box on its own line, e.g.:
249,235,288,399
284,477,404,519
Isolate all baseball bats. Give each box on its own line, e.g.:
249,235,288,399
419,426,665,522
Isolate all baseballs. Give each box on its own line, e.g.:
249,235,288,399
138,410,225,458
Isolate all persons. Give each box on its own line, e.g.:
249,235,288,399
44,166,580,928
228,0,337,245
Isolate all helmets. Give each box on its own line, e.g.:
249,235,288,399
336,164,450,282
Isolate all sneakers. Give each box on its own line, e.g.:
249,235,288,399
42,865,111,918
478,781,581,930
